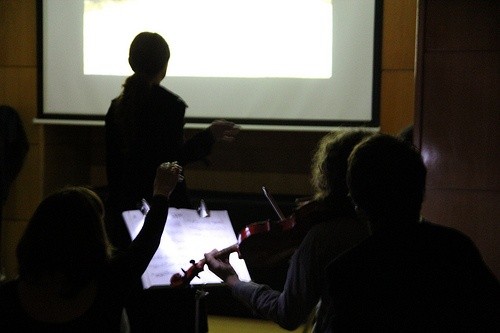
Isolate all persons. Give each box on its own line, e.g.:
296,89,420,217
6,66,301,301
0,160,184,333
323,134,500,332
104,33,235,332
204,129,375,330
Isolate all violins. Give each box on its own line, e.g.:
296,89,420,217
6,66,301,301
170,197,339,287
